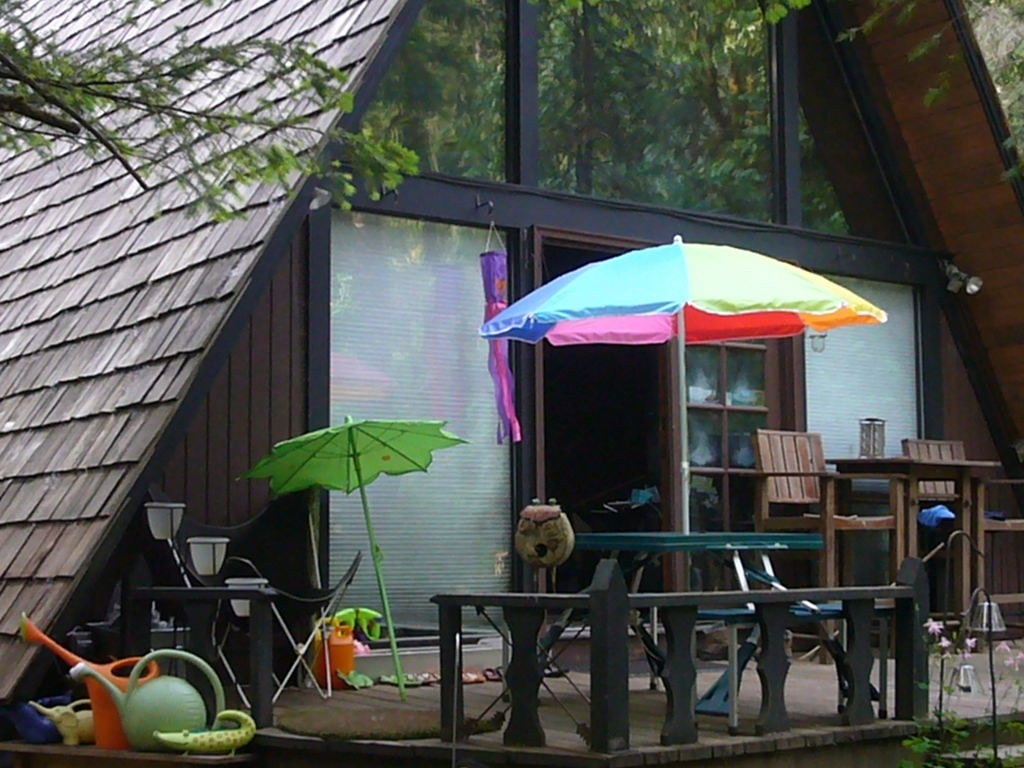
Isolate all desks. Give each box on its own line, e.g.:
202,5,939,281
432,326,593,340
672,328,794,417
573,532,825,706
815,457,1003,655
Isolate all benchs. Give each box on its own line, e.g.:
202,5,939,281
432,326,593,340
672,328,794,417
693,602,898,714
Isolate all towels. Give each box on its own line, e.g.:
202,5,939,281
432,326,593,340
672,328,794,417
918,504,956,527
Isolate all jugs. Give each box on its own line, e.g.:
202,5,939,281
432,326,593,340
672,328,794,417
19,612,225,753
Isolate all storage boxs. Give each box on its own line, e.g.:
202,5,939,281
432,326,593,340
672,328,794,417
90,617,190,682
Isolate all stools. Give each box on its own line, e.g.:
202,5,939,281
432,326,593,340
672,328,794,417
897,437,1024,644
751,426,909,663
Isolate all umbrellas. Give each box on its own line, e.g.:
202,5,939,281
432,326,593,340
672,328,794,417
236,415,473,702
479,232,891,719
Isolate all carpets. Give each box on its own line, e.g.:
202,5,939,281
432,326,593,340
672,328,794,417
278,708,500,738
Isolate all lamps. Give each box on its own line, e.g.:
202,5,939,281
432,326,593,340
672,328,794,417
144,500,184,540
223,578,268,618
185,536,231,575
309,187,333,211
968,601,1006,635
941,257,986,295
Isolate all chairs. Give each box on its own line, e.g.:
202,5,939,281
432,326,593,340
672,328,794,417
145,484,363,710
